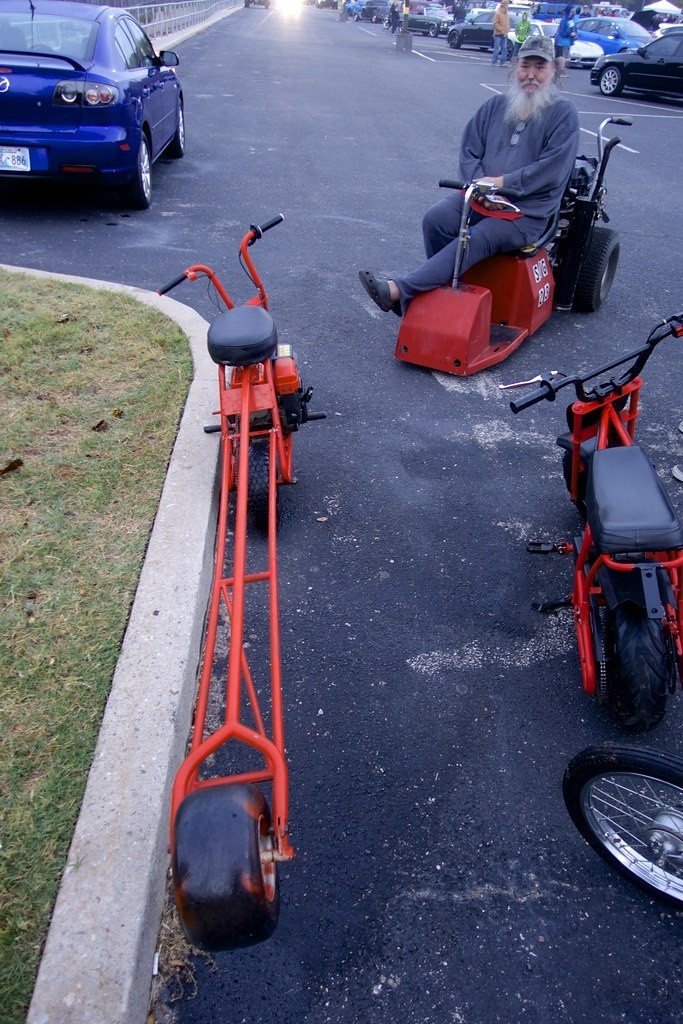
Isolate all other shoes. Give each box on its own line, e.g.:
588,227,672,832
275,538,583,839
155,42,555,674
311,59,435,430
358,269,394,312
493,63,507,67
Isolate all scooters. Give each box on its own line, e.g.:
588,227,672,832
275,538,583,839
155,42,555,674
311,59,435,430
497,312,683,731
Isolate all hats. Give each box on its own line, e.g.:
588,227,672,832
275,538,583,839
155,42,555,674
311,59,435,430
517,34,556,62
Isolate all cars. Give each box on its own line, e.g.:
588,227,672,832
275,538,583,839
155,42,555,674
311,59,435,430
0,0,187,209
446,11,531,49
500,22,605,70
573,16,655,55
303,0,683,67
589,30,683,96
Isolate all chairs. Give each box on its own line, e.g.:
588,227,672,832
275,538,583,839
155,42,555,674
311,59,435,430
0,17,26,51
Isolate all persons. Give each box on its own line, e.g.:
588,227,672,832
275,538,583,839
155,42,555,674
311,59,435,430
655,13,683,31
491,0,510,66
390,0,400,36
576,5,628,18
528,2,543,16
452,0,468,25
336,0,364,22
508,12,532,68
359,36,580,318
480,0,487,9
554,5,579,77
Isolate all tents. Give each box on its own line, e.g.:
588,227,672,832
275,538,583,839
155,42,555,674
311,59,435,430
642,0,682,16
